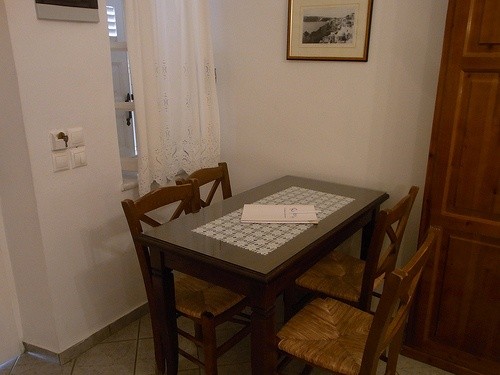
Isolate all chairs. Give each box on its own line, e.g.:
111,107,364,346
276,225,443,375
122,162,297,375
297,186,419,360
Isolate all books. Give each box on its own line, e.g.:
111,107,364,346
241,203,318,225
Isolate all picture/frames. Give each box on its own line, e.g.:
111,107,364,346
286,0,374,62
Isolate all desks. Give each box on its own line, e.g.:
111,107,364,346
137,175,390,375
